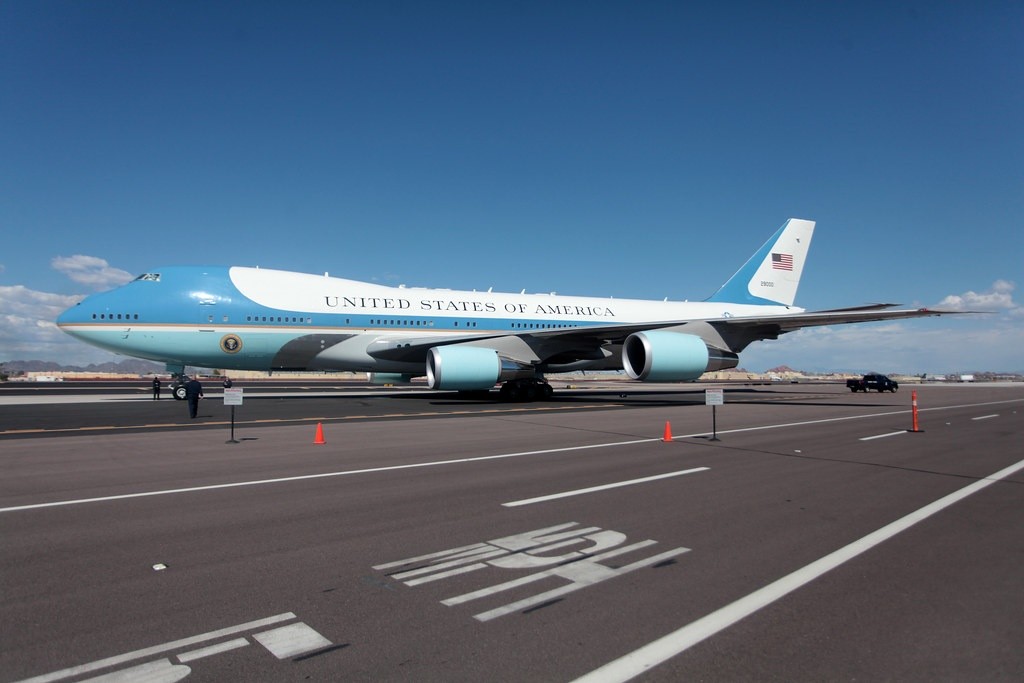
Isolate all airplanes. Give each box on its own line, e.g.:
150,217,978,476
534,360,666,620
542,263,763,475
56,218,999,392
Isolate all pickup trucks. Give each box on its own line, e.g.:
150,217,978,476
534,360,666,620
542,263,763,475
846,374,899,392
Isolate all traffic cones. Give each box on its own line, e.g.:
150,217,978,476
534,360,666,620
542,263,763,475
663,420,674,442
312,423,326,445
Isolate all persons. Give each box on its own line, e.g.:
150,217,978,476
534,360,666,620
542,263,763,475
186,374,204,419
152,377,161,400
223,377,232,388
